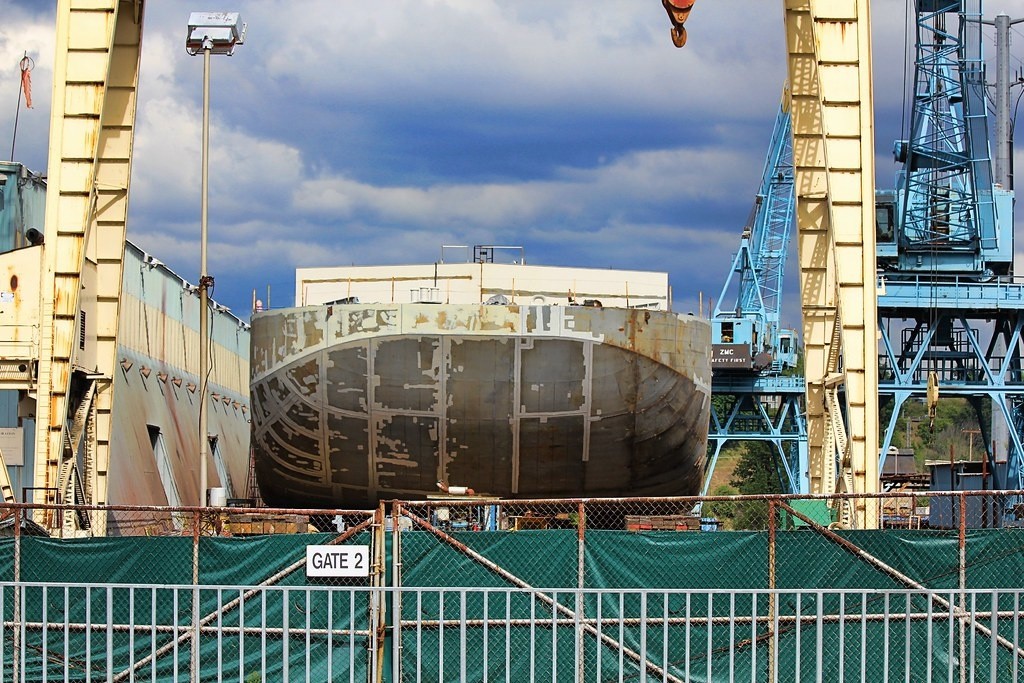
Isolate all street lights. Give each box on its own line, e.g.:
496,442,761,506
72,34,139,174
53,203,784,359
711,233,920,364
187,11,248,508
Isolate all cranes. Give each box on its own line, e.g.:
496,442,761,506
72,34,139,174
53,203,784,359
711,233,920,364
659,0,1023,532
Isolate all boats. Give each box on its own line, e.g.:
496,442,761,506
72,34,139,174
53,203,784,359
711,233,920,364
241,238,718,532
